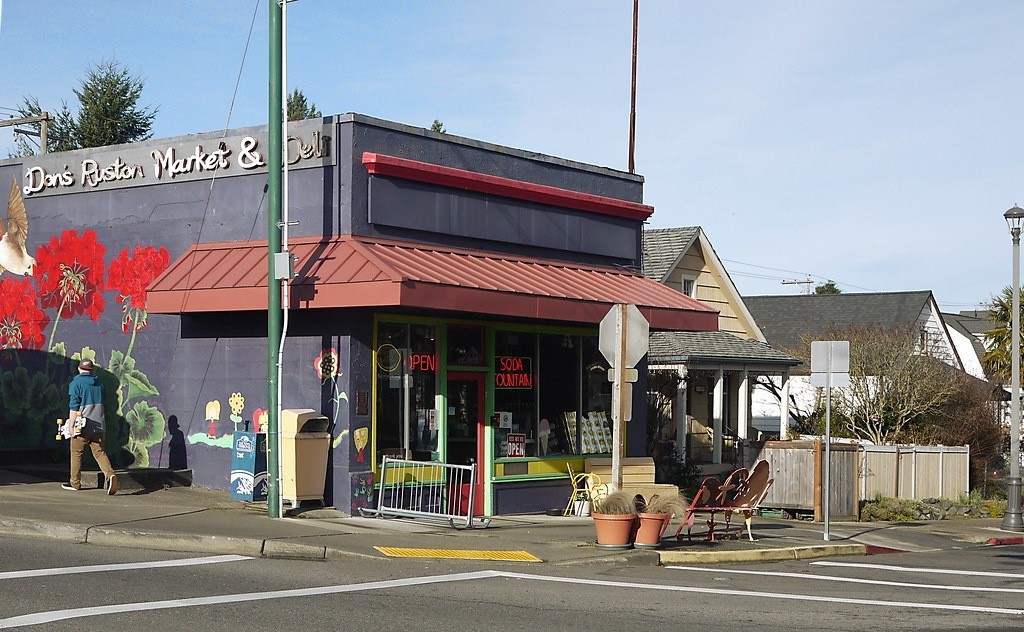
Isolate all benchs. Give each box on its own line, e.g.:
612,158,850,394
373,462,447,489
660,459,774,542
584,457,678,499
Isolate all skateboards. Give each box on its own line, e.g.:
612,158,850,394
55,411,86,441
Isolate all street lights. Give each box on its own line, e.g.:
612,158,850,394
999,202,1024,534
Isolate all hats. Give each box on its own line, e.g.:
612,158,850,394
79,358,94,370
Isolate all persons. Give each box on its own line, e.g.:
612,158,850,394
61,359,118,496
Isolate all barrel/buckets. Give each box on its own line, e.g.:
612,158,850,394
574,500,590,515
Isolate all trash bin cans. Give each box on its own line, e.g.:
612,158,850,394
281,408,331,509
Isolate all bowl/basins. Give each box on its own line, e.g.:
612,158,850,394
545,509,563,516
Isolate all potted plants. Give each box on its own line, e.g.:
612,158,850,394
633,489,692,543
574,492,590,517
591,491,637,545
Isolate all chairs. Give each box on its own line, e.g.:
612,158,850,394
563,461,608,517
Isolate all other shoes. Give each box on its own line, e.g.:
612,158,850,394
106,474,118,495
61,484,78,491
